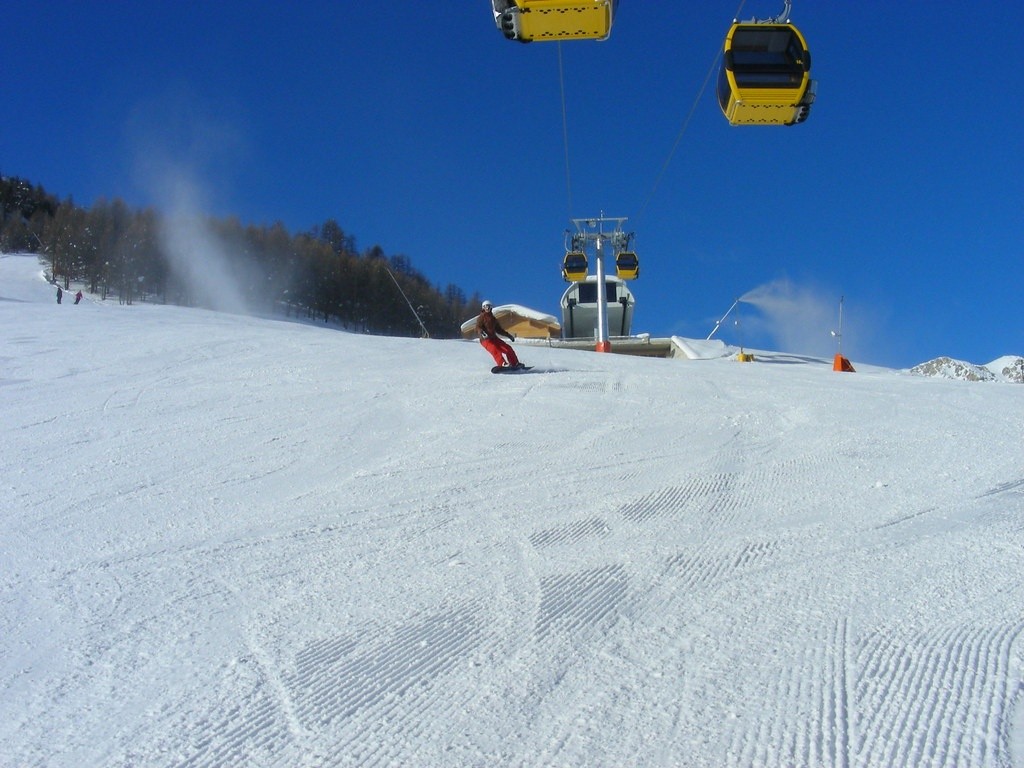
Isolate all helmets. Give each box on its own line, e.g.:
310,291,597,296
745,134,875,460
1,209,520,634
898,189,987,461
482,300,493,311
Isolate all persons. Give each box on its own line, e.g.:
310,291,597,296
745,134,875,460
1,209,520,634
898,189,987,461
56,288,62,304
74,290,82,304
476,300,525,367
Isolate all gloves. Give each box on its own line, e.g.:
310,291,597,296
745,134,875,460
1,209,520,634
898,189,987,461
509,335,515,342
481,330,489,339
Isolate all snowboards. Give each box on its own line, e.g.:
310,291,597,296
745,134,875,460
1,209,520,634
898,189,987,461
490,365,536,375
